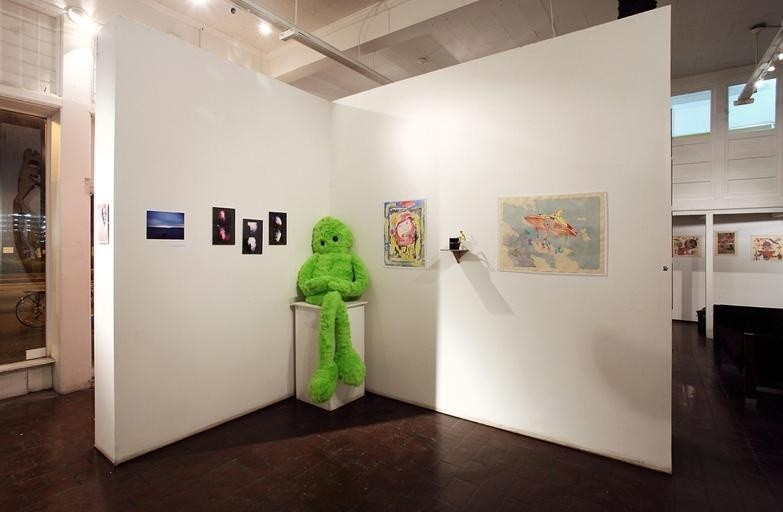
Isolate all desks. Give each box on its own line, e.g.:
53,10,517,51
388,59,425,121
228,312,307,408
292,300,368,413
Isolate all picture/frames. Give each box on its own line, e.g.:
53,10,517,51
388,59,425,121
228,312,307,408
713,229,739,257
497,192,609,277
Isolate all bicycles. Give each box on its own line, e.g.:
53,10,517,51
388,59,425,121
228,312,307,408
15,289,45,328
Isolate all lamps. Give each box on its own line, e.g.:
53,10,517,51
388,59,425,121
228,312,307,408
733,23,783,107
